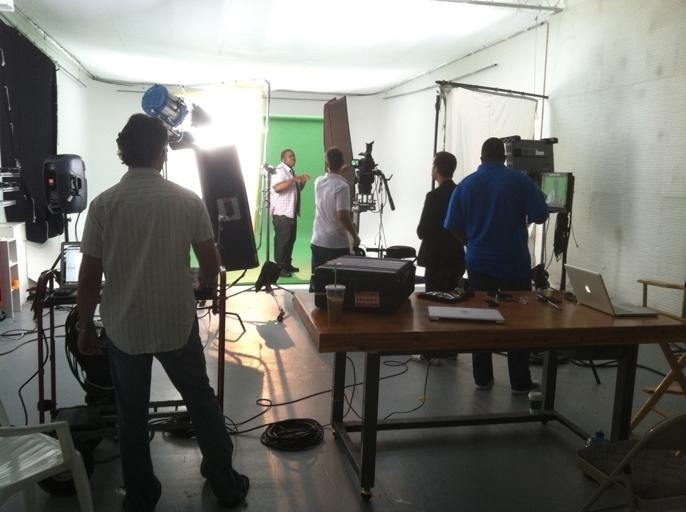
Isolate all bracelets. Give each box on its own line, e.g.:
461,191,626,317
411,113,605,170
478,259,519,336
75,321,92,333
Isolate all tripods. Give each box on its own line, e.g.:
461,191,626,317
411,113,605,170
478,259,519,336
226,163,294,323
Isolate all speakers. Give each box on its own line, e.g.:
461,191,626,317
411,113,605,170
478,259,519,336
42,152,86,215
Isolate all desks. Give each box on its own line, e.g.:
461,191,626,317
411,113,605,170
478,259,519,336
291,287,686,500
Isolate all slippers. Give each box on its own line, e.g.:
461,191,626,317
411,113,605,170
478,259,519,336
218,474,247,507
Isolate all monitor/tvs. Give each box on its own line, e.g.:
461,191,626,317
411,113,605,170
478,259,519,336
540,173,573,209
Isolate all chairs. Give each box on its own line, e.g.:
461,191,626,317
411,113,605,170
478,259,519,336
0,400,95,511
576,412,686,511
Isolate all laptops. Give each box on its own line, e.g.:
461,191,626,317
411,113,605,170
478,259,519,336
564,264,659,317
50,241,106,299
427,305,505,324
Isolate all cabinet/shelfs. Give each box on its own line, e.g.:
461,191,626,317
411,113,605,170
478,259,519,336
0,222,30,318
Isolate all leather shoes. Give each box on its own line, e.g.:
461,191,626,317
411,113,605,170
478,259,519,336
279,267,291,277
284,264,299,272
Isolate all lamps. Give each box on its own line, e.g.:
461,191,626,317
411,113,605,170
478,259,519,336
141,85,212,154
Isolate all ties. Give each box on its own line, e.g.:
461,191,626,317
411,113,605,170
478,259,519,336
291,168,300,217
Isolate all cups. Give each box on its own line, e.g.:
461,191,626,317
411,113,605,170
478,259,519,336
325,283,347,324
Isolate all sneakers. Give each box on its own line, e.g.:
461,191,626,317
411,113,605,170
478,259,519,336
475,376,494,390
511,380,540,394
410,354,441,366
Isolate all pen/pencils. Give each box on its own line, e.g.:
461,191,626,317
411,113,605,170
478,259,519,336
537,294,561,310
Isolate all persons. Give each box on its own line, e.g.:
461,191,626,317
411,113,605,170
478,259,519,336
442,136,549,397
269,149,311,278
74,114,254,511
309,145,362,295
410,150,467,367
